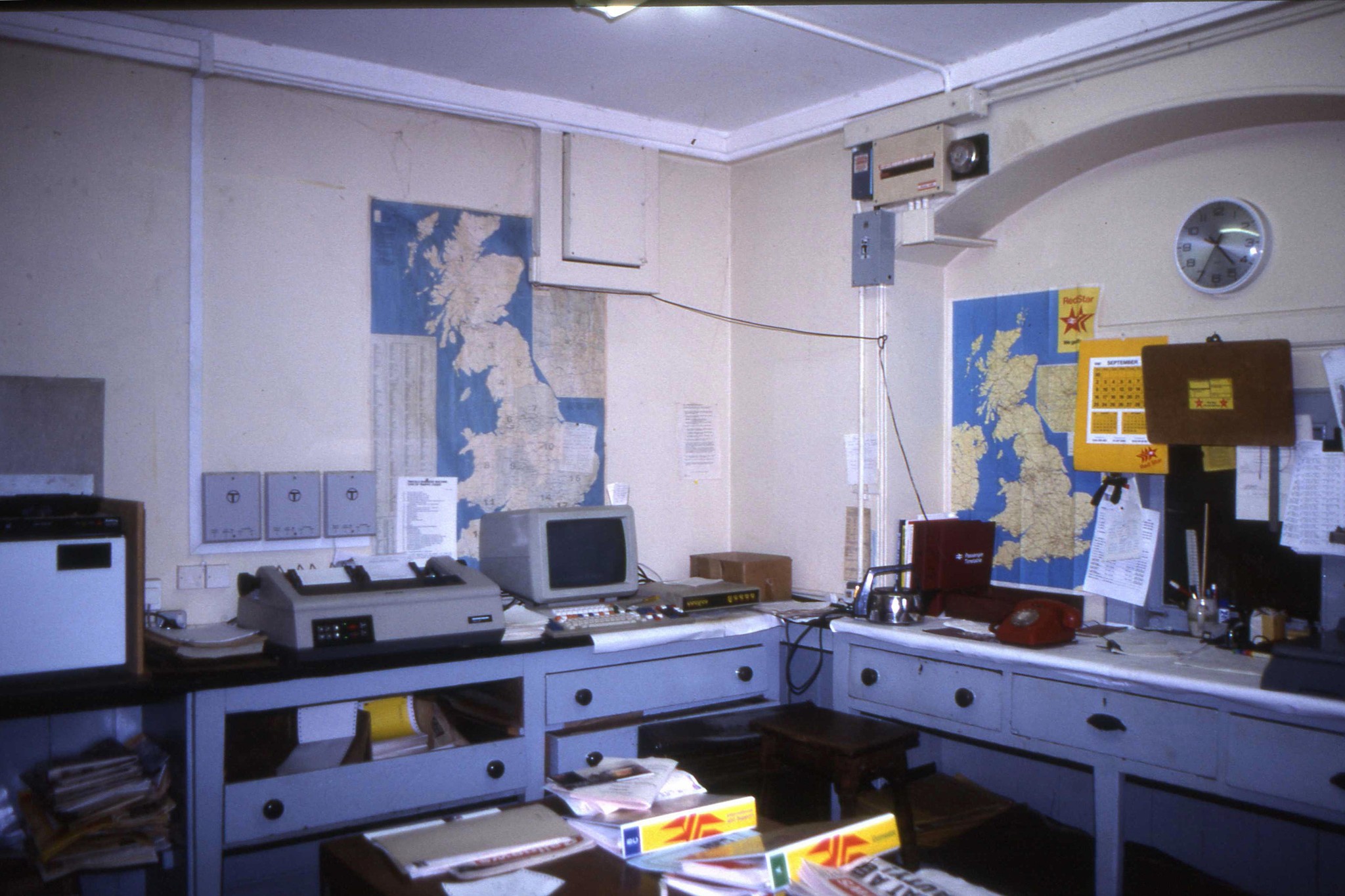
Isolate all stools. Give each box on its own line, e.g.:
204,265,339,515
748,710,920,874
855,769,1030,892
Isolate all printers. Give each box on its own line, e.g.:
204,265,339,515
236,556,507,663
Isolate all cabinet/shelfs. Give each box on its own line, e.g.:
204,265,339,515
184,619,778,896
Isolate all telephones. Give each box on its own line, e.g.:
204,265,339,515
998,598,1079,648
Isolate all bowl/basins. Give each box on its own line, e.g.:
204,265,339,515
867,586,924,625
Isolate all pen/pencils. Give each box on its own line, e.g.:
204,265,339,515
1169,580,1217,598
1231,648,1272,659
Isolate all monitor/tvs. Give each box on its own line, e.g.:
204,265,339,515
480,505,638,607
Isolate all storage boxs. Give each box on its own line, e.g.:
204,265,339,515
689,552,793,603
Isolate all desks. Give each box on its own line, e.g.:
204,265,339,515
317,797,1003,896
830,613,1345,896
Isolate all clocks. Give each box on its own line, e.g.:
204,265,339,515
1175,197,1269,294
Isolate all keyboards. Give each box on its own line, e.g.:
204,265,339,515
542,602,694,637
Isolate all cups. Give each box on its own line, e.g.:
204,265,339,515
1187,598,1218,637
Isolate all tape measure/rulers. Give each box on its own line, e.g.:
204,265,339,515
1186,529,1200,596
